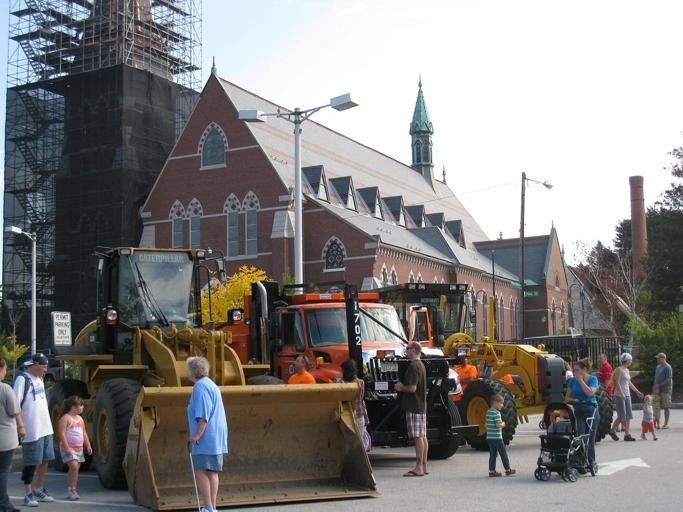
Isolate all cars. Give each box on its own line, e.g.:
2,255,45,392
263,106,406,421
44,357,79,382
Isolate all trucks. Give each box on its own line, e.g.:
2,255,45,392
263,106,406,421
203,284,479,460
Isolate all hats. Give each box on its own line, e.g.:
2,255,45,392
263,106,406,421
654,353,666,359
24,353,48,365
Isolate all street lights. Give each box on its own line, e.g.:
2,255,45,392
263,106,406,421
239,94,358,296
541,300,565,337
4,225,37,365
520,171,552,342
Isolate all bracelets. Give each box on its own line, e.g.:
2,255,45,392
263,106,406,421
17,424,24,429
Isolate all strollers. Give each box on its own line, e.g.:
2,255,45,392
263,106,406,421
535,401,598,482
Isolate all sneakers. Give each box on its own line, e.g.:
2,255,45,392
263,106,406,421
505,469,515,475
624,435,635,440
609,431,620,440
489,471,501,476
24,492,38,506
33,488,54,501
69,488,80,500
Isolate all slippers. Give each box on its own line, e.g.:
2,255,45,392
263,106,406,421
403,471,423,476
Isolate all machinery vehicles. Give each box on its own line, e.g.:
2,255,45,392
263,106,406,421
47,242,383,512
369,283,613,451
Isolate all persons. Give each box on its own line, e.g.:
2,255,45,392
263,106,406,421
184,355,229,512
491,359,515,384
58,395,93,500
542,351,644,464
394,341,430,477
640,394,659,441
654,352,673,429
12,353,55,507
0,357,21,512
336,359,371,453
485,394,518,476
447,356,479,406
650,383,661,429
286,354,316,384
